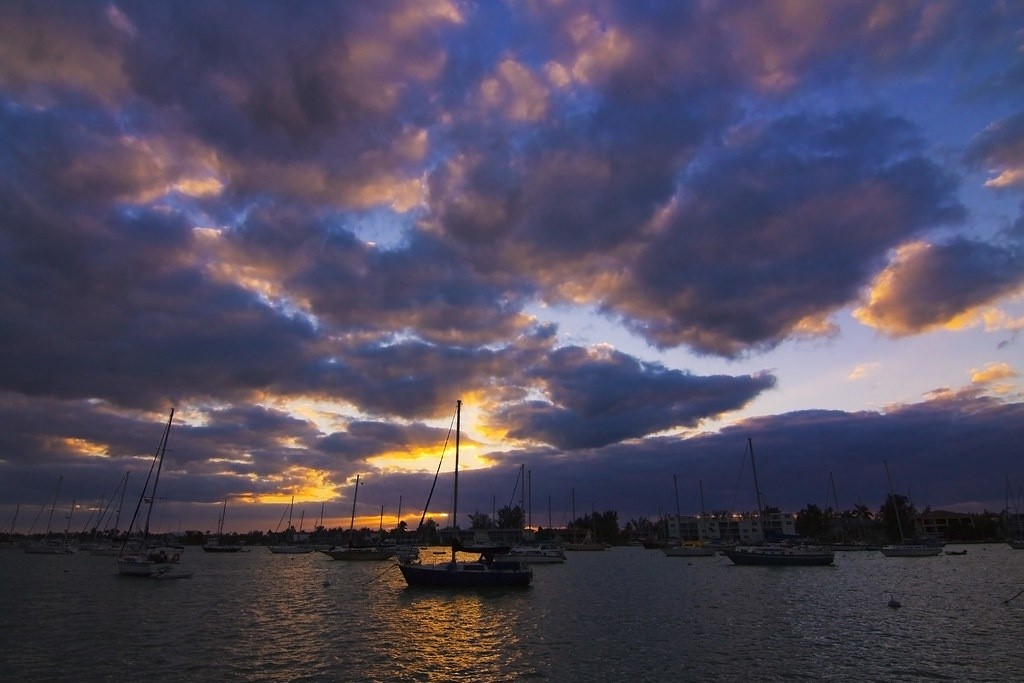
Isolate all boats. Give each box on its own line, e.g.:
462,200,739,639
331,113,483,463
640,537,678,551
496,546,569,565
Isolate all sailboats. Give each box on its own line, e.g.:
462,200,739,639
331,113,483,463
400,399,535,590
803,468,882,551
559,486,610,552
725,437,837,567
664,480,718,558
115,406,202,579
884,456,944,561
0,470,143,555
203,496,246,554
267,473,518,561
